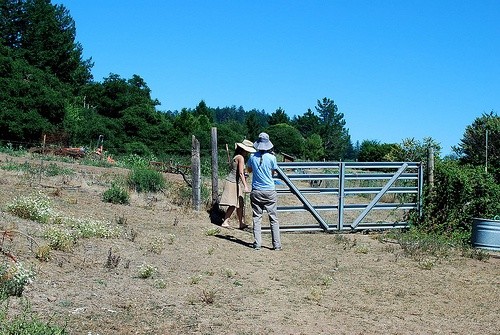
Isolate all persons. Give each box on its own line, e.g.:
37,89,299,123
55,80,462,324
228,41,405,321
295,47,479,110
246,132,282,250
218,139,256,229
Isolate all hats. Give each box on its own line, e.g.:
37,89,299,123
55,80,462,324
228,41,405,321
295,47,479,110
236,139,256,152
253,133,273,150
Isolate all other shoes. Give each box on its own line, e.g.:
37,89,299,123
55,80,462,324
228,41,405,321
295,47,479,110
274,246,282,251
253,245,261,250
221,224,234,230
240,224,249,229
